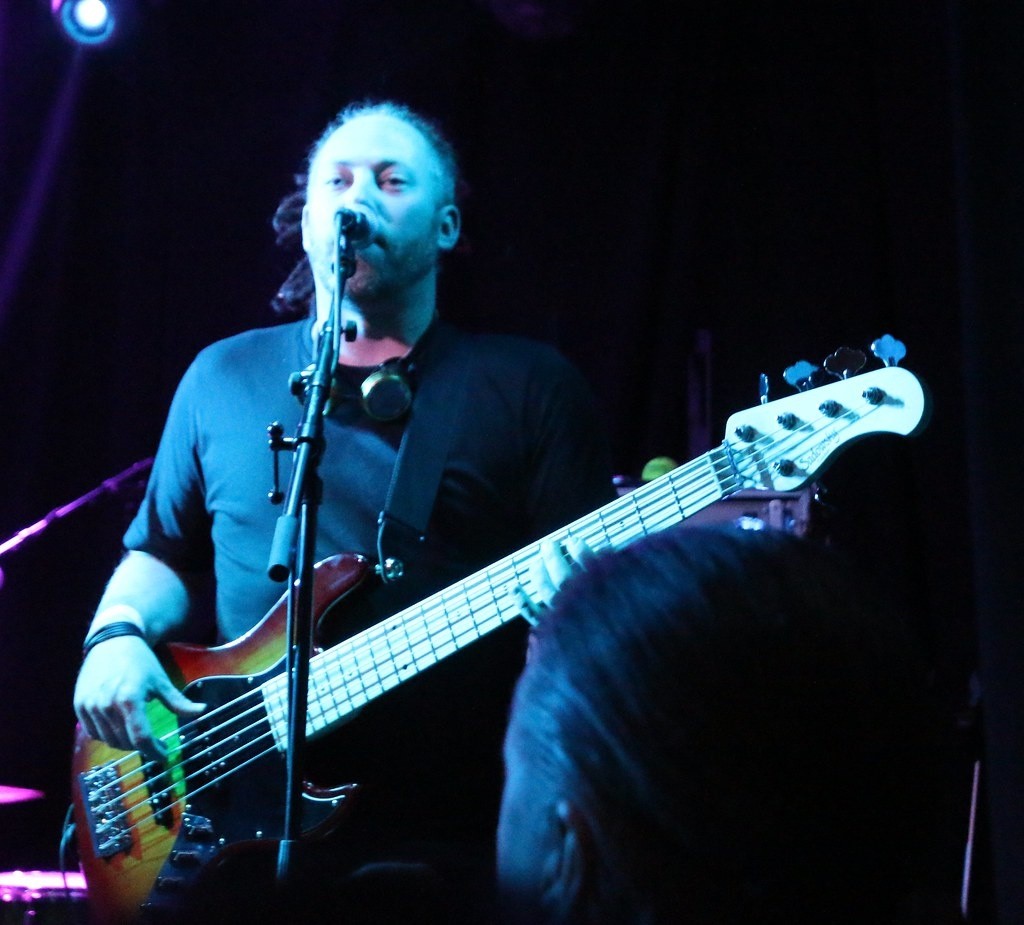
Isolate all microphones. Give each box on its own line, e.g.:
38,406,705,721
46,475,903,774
334,208,380,251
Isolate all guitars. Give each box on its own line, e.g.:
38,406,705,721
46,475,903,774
70,332,925,922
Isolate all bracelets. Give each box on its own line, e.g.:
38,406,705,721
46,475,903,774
82,622,145,656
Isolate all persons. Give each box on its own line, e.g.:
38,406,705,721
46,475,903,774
499,528,936,925
70,98,595,840
